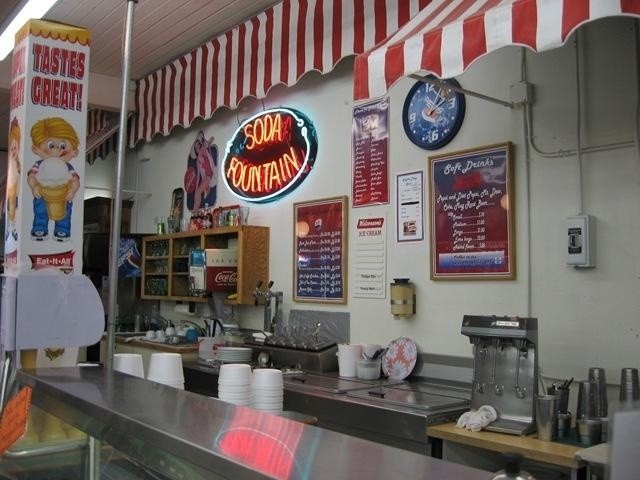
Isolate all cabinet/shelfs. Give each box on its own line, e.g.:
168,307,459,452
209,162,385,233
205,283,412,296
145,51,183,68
140,224,271,306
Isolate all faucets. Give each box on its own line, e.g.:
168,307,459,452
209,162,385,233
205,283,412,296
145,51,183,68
180,319,204,337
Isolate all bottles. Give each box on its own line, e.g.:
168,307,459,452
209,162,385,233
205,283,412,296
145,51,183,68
167,209,177,234
189,203,212,232
233,213,241,226
216,205,225,228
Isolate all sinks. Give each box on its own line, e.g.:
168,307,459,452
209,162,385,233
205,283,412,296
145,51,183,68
116,332,187,345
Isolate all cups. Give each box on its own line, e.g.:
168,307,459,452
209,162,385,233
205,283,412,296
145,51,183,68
577,380,598,420
548,387,571,440
576,420,602,447
588,368,608,417
534,396,560,443
166,327,175,336
619,368,639,403
156,330,165,339
146,331,155,338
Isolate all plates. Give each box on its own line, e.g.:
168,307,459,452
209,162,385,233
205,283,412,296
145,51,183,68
216,347,252,362
383,338,417,382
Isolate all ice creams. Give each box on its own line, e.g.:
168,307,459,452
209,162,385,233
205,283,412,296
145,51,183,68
6,160,19,220
35,157,70,222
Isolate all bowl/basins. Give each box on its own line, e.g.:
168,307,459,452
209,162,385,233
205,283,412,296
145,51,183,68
114,354,144,378
252,369,283,412
147,353,184,386
357,359,381,380
218,364,252,404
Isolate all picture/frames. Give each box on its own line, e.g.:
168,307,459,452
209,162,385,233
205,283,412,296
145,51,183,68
292,194,350,306
426,140,519,281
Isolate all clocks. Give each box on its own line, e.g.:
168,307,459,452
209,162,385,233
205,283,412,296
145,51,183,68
401,73,467,151
